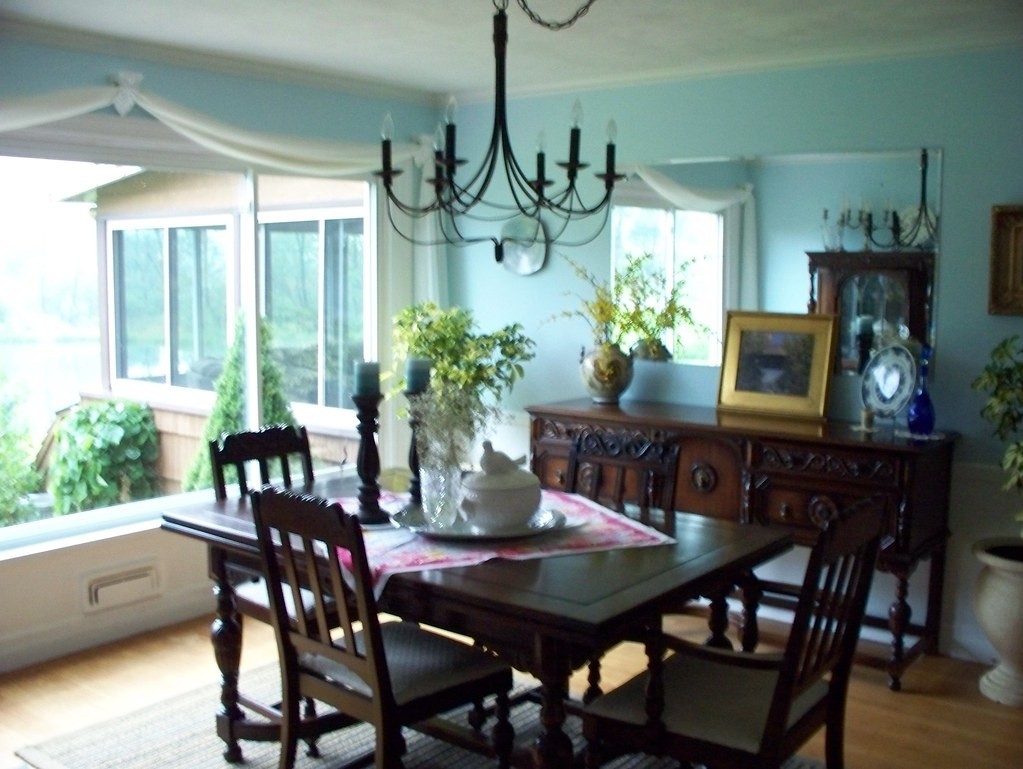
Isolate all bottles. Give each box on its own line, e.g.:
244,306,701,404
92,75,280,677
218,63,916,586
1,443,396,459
905,341,937,436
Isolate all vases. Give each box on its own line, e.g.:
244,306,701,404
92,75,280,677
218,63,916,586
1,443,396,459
628,339,672,360
580,343,636,404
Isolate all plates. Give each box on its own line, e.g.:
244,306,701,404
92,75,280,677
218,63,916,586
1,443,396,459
860,346,916,419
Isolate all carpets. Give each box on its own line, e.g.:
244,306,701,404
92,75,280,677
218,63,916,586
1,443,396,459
13,661,716,769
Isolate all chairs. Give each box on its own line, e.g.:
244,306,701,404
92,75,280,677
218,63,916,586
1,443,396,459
208,425,888,769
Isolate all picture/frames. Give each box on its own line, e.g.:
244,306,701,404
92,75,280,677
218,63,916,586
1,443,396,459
717,310,837,420
988,203,1023,317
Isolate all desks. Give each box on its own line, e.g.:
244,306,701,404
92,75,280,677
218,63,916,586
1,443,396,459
160,464,795,769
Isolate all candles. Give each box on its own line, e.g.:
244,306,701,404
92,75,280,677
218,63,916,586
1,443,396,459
407,359,432,391
353,360,380,395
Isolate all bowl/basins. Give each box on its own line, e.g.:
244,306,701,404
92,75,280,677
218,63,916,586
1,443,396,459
456,440,543,532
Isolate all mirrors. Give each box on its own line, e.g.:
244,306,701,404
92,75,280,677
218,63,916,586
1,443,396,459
607,146,945,375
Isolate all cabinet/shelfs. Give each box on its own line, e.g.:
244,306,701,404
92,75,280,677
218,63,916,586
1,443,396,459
525,399,966,693
806,250,935,369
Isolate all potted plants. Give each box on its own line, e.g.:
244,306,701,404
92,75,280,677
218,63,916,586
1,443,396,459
972,332,1022,708
385,304,536,528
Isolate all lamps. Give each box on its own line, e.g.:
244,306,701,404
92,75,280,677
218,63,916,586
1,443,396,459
370,1,627,263
820,147,941,253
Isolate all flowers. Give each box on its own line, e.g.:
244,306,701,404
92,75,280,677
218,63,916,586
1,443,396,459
538,248,687,357
607,256,717,357
